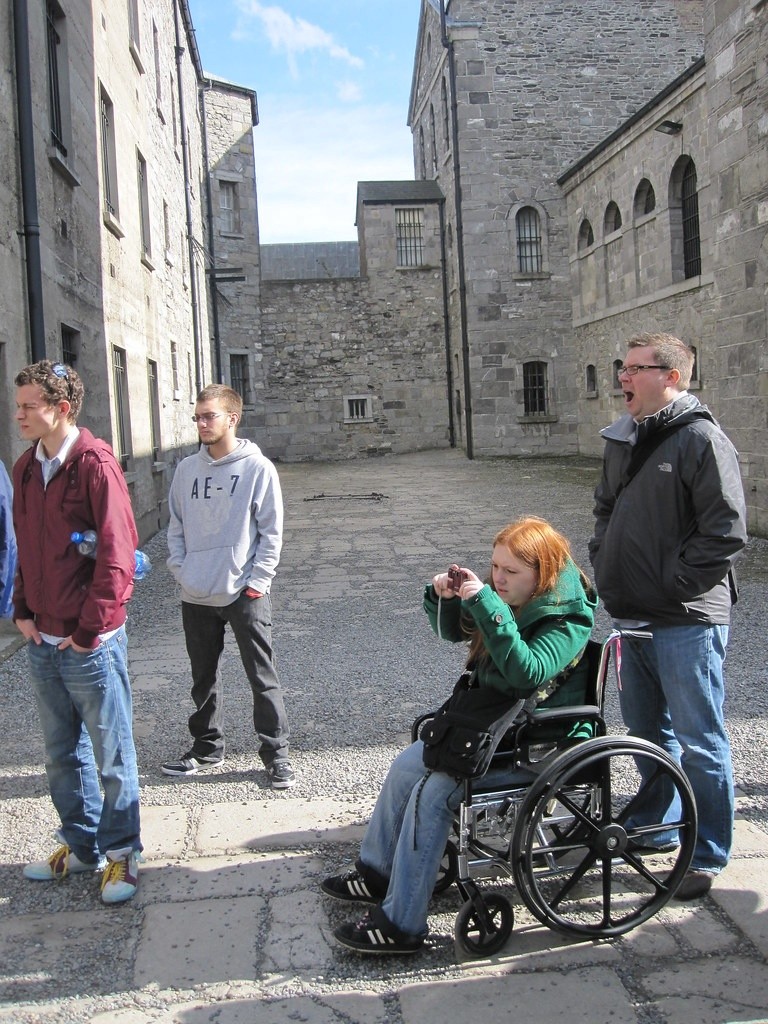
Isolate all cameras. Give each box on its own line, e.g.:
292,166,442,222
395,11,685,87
447,568,469,592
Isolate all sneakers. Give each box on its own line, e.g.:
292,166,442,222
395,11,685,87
321,872,383,904
23,846,107,879
334,910,423,953
160,752,225,776
100,847,140,903
266,762,297,788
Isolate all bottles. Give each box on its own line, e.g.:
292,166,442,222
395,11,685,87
71,530,152,579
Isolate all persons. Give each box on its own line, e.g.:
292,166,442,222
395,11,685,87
319,517,599,951
588,334,749,901
0,460,18,620
10,362,144,903
161,384,294,787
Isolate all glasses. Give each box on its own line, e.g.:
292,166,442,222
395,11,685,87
617,365,666,376
192,412,232,422
51,362,73,401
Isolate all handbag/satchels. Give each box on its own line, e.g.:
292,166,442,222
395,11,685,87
420,672,531,779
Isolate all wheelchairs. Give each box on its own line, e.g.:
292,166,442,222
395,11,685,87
434,628,699,959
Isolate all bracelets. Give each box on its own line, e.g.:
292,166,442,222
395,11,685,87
245,591,263,598
